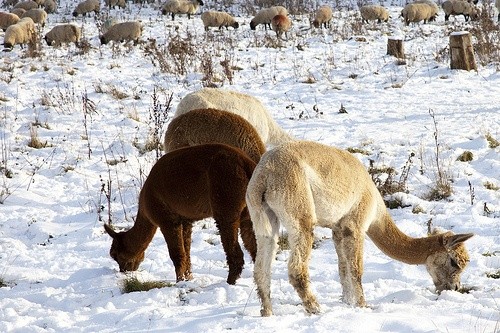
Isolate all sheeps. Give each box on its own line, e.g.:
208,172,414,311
44,0,61,14
247,5,292,40
99,21,144,46
44,24,82,45
442,1,481,23
246,139,475,316
361,5,390,24
202,11,241,31
164,108,269,162
161,0,204,21
96,142,259,287
402,0,438,25
312,6,333,29
175,90,306,146
70,0,102,18
0,1,48,48
